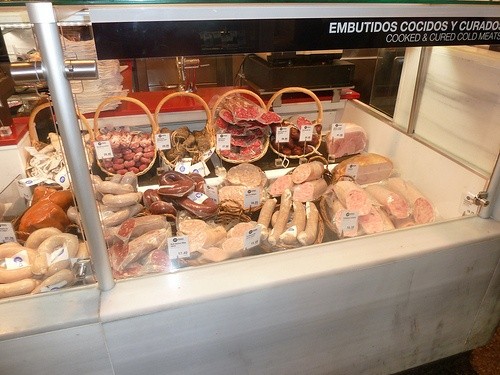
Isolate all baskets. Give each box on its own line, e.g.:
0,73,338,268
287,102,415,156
26,88,323,189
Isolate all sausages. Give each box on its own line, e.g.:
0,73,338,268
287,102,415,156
0,161,435,298
97,127,210,173
269,123,323,156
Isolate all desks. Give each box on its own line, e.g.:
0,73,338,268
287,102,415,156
78,81,360,119
